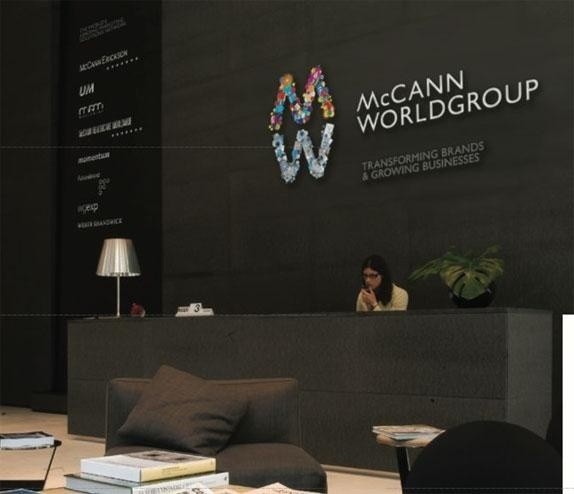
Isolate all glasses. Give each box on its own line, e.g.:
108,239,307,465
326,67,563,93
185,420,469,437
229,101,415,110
363,272,379,279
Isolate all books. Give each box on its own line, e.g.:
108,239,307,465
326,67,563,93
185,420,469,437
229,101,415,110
242,479,317,493
61,469,229,494
0,430,53,451
371,422,442,441
79,448,216,484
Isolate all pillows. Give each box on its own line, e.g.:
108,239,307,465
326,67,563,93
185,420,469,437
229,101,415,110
114,363,254,459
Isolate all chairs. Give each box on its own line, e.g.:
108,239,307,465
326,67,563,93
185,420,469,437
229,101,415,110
404,420,562,494
104,377,329,494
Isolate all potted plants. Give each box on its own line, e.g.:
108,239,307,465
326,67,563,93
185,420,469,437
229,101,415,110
407,242,506,308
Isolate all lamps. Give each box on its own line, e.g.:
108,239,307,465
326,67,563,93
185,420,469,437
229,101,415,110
95,237,143,318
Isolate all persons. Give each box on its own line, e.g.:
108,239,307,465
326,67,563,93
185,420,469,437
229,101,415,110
354,253,409,313
